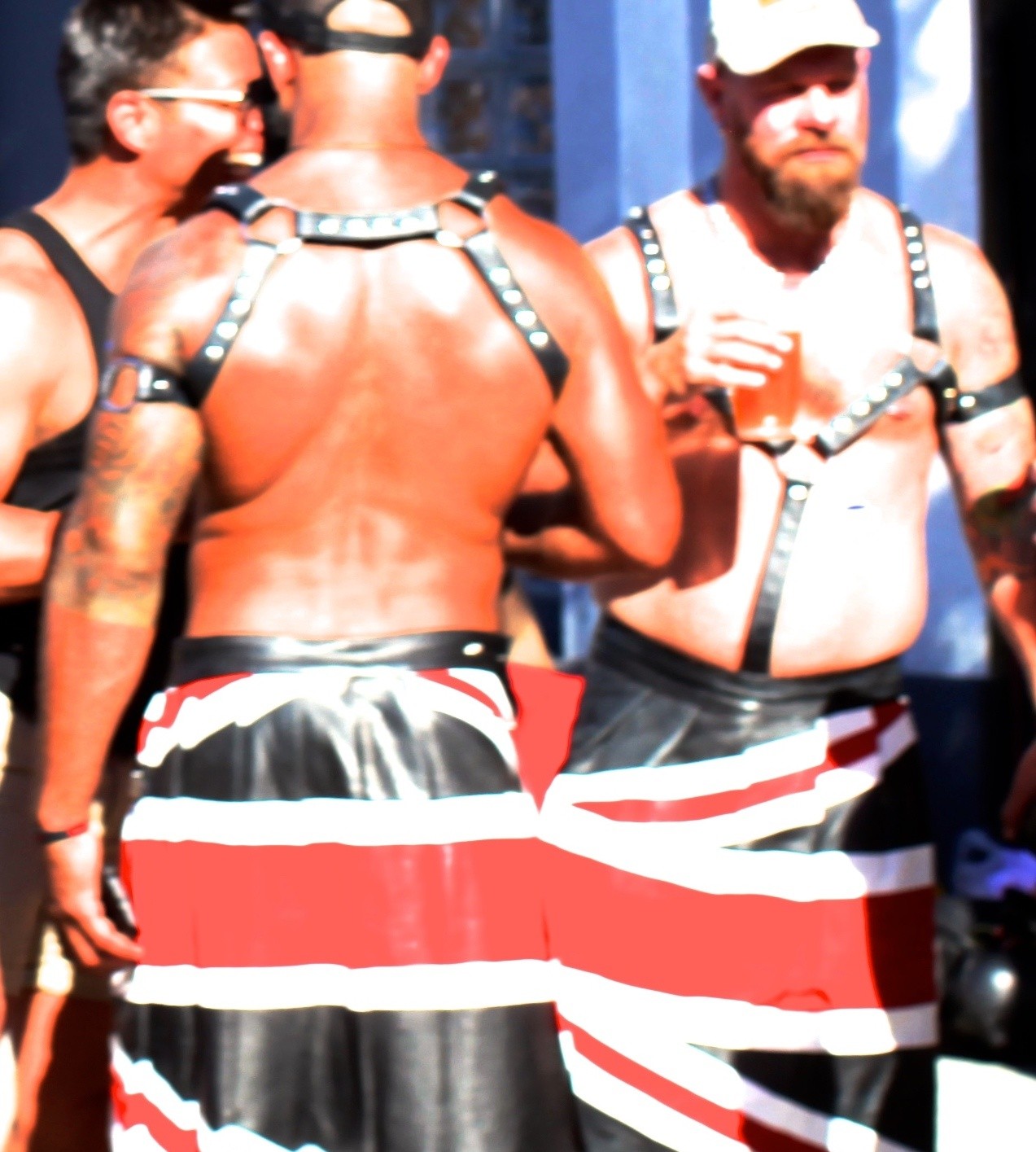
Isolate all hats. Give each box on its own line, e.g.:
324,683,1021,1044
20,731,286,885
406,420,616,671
261,0,437,62
710,0,880,79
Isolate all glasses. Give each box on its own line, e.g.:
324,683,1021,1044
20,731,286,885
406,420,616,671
121,77,276,111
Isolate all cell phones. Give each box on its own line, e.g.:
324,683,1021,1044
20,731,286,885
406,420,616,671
99,864,140,940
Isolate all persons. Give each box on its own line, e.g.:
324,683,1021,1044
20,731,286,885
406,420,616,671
30,1,682,1151
1,0,1036,1152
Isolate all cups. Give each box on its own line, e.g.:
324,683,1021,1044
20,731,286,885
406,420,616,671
727,294,808,443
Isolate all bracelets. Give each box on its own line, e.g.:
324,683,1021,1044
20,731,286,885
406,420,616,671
24,820,91,847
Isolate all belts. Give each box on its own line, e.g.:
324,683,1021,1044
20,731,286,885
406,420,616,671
587,616,908,701
164,629,513,688
